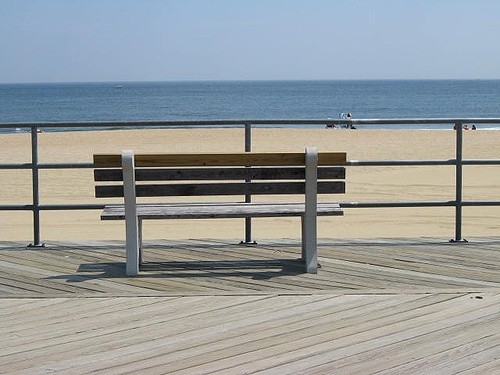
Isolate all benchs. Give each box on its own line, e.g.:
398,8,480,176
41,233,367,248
92,146,347,277
